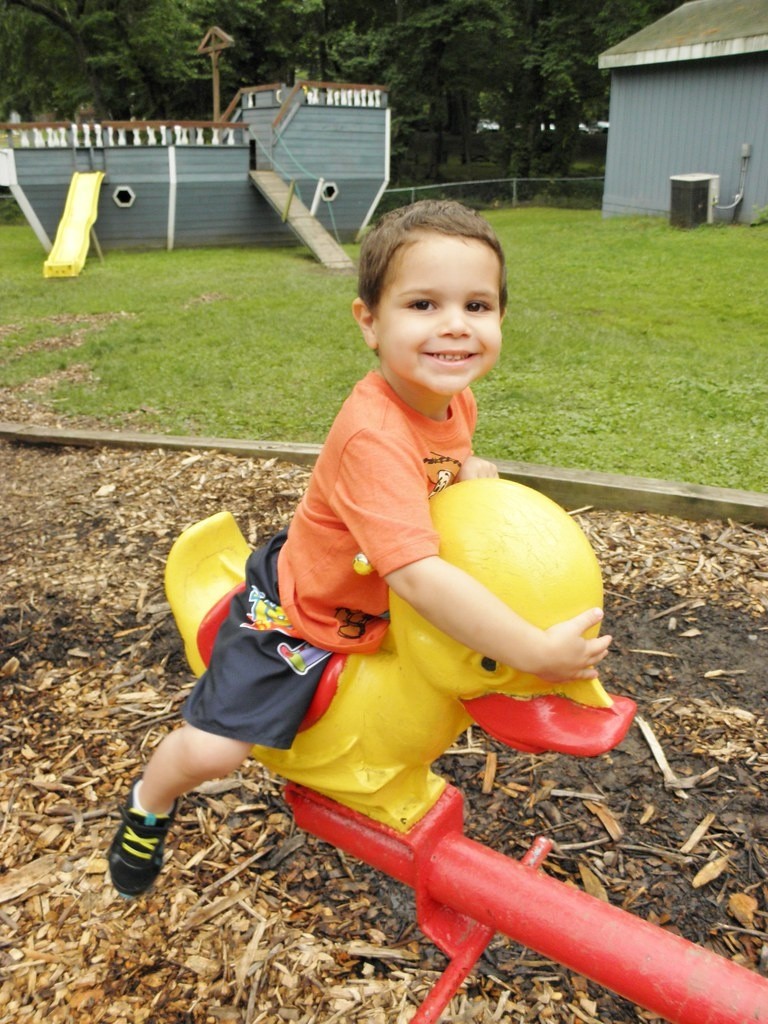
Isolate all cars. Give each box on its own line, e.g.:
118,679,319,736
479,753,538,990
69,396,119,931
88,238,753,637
476,119,501,133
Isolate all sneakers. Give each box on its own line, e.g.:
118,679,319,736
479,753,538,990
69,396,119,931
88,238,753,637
108,776,179,897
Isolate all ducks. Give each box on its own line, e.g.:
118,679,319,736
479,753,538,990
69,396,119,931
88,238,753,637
164,477,639,834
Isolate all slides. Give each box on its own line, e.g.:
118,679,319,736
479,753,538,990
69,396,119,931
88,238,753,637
41,170,105,278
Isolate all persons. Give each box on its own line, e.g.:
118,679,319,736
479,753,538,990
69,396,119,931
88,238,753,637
107,198,613,900
